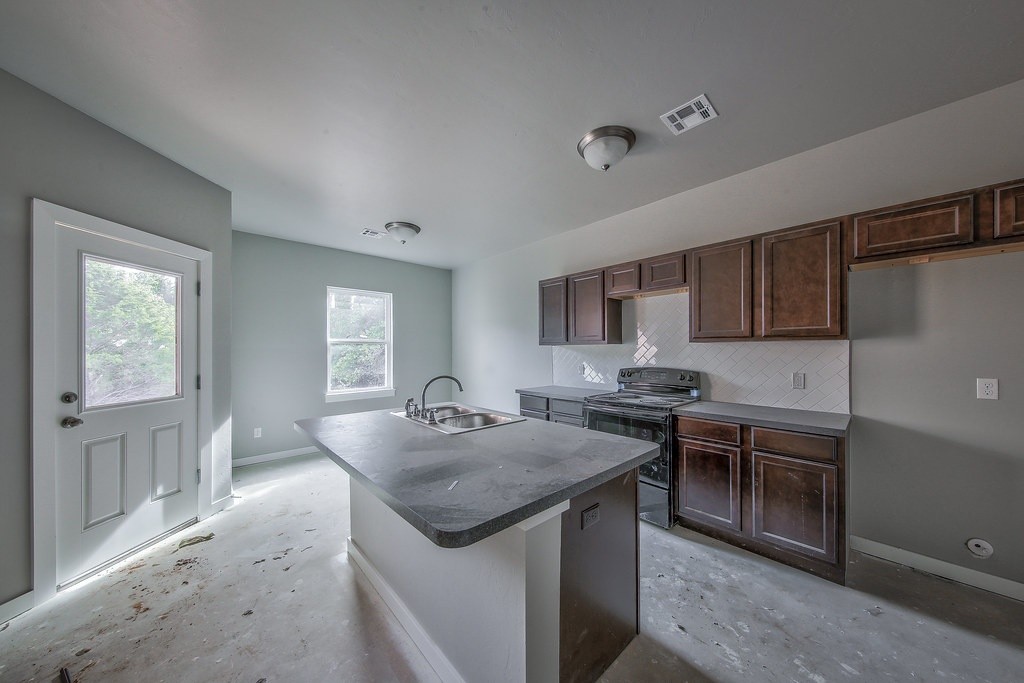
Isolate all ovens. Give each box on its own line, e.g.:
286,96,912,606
582,404,679,530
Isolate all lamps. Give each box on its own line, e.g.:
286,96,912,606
577,125,636,171
386,222,420,244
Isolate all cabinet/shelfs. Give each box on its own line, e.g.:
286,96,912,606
538,268,623,345
520,394,586,428
604,251,690,300
672,414,846,587
689,215,848,342
848,177,1024,272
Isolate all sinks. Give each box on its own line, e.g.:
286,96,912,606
433,413,527,435
390,402,476,420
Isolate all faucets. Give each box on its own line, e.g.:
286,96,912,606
421,375,464,417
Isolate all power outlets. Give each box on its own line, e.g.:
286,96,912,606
254,428,261,438
793,372,805,389
976,377,998,400
581,503,600,528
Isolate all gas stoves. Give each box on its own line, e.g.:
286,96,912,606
585,368,701,413
